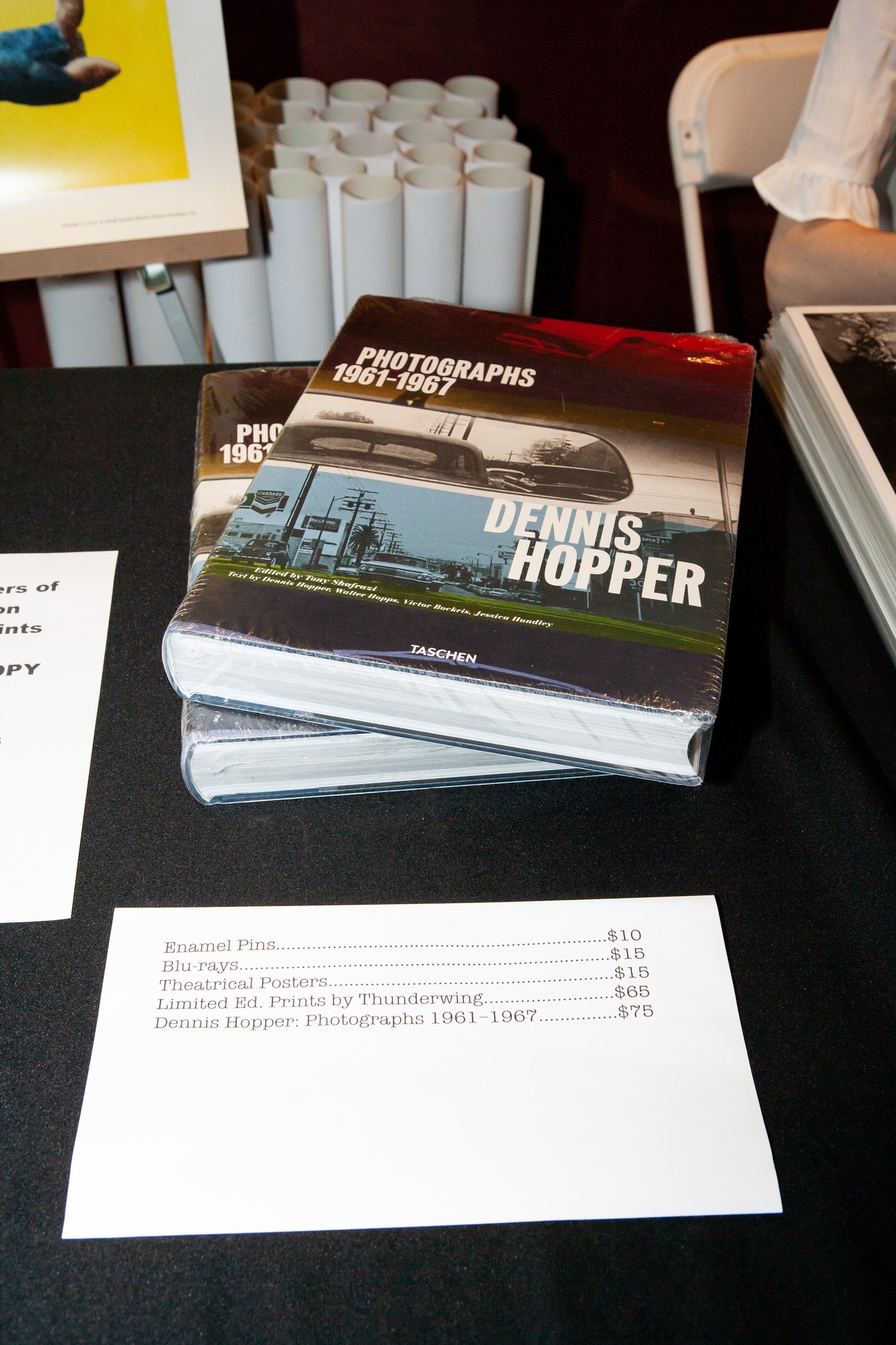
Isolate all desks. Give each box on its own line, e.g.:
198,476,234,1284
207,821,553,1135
0,350,896,1345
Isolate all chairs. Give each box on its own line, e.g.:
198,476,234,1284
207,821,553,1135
662,27,836,337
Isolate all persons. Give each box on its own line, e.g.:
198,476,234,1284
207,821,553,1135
750,0,896,324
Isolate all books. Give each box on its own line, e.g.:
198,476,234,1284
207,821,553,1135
181,363,619,806
160,293,759,789
